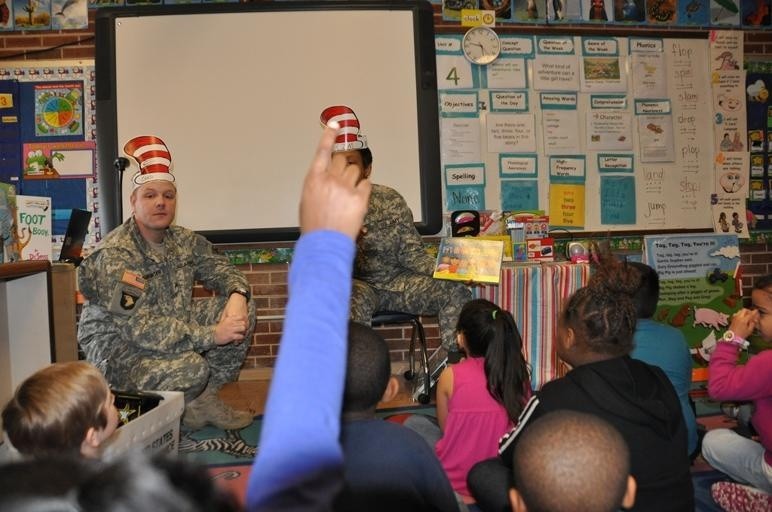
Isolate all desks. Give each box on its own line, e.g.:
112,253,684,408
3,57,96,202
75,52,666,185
466,262,590,390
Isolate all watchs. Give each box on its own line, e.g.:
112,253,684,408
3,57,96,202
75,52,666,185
722,331,749,351
227,285,251,303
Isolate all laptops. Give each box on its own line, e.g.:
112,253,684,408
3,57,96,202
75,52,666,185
51,207,93,265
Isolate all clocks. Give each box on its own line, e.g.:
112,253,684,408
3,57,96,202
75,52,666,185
460,28,501,65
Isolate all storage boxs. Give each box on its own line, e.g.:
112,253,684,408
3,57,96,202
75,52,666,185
104,388,185,457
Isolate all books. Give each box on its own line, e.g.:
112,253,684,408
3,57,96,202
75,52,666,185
432,237,503,286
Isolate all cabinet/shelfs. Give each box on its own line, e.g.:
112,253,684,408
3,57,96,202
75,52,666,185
51,262,78,364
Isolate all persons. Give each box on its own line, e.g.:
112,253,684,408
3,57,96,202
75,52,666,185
505,410,638,512
319,104,473,364
80,455,245,511
340,320,461,511
1,360,120,459
76,136,253,433
243,119,441,512
436,297,534,496
701,272,771,512
618,262,701,466
0,458,82,512
467,284,696,511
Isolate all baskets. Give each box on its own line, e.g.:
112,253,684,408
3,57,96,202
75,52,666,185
2,390,185,462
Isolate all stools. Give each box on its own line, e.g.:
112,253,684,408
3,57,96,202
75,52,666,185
373,312,431,404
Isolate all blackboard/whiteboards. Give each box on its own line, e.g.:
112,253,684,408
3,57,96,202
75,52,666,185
0,24,752,253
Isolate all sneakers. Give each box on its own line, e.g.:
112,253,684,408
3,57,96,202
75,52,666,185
179,383,253,432
711,482,772,512
